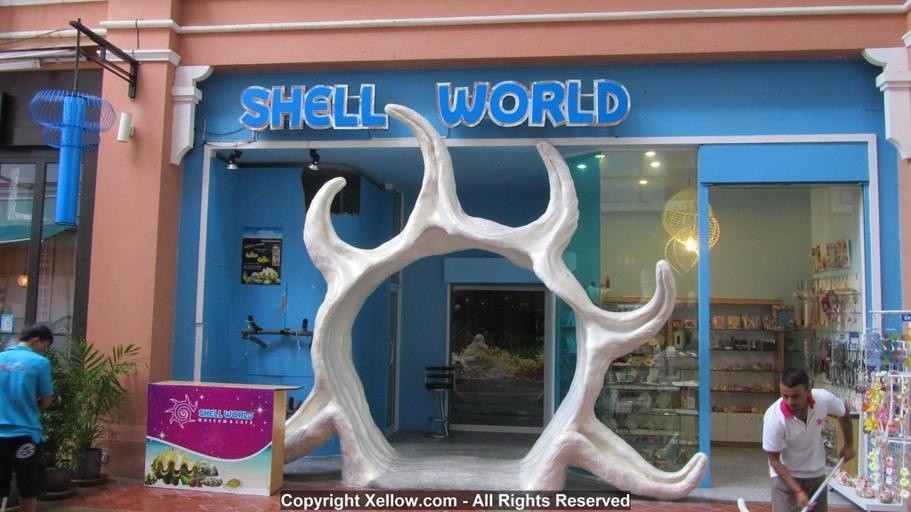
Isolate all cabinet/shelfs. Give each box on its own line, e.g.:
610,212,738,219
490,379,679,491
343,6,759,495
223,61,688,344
601,295,785,448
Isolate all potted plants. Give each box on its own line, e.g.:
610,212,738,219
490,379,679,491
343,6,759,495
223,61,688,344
27,333,141,496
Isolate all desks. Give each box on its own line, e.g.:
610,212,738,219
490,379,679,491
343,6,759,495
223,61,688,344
142,381,302,497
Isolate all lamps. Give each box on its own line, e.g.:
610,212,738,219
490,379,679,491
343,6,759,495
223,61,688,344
223,148,241,170
29,27,132,227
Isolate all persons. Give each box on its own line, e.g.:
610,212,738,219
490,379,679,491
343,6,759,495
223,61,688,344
647,332,697,459
0,322,55,512
761,367,856,512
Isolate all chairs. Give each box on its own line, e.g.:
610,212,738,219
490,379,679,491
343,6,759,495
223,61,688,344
422,365,456,440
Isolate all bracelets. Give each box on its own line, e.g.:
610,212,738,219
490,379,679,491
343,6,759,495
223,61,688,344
791,487,803,496
